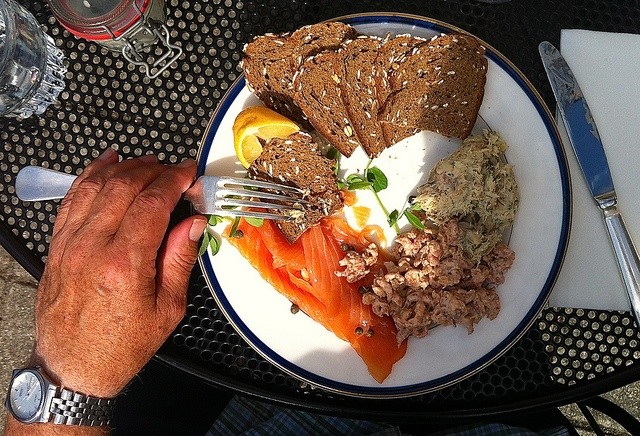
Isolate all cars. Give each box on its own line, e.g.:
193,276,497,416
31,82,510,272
0,0,69,123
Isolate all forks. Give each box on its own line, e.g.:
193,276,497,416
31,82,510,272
14,165,307,222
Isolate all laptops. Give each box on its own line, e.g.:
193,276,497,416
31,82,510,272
240,22,488,158
247,131,343,243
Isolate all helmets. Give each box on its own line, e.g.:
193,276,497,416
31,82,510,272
189,10,574,401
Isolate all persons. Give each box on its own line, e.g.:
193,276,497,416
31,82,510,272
0,147,592,435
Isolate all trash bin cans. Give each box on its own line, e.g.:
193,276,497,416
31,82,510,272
3,363,116,429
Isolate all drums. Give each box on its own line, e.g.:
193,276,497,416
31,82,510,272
538,41,640,332
